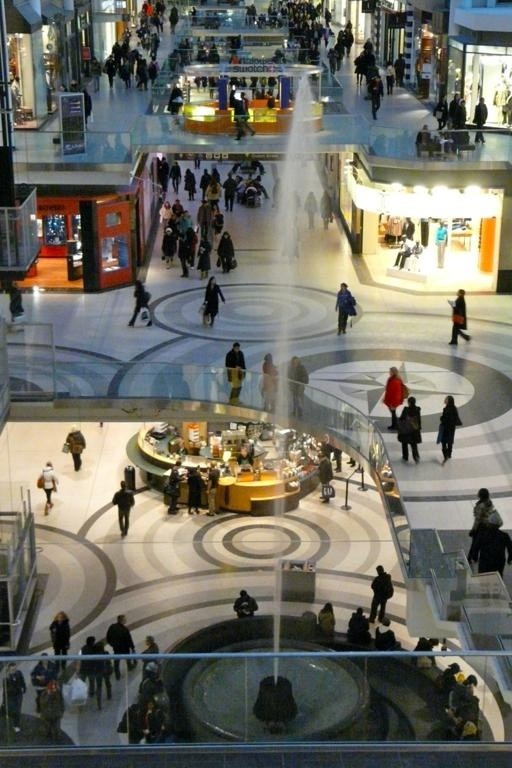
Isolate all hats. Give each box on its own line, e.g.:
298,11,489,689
166,227,172,233
489,510,502,526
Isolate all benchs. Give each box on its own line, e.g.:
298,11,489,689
417,130,475,159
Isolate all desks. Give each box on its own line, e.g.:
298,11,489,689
452,230,473,248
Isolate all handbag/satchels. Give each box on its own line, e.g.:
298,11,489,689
63,443,71,453
38,477,44,488
453,314,464,324
230,260,236,269
397,416,420,435
217,257,221,267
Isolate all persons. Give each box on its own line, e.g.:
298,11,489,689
233,590,259,618
468,488,511,579
67,425,86,471
224,341,310,421
317,566,484,744
334,283,354,337
392,218,449,273
165,430,253,517
271,151,334,263
6,282,25,322
381,367,421,465
316,440,356,503
65,2,510,158
41,460,58,516
0,609,173,745
121,152,272,329
448,289,471,346
437,395,462,462
9,71,21,125
112,482,135,537
